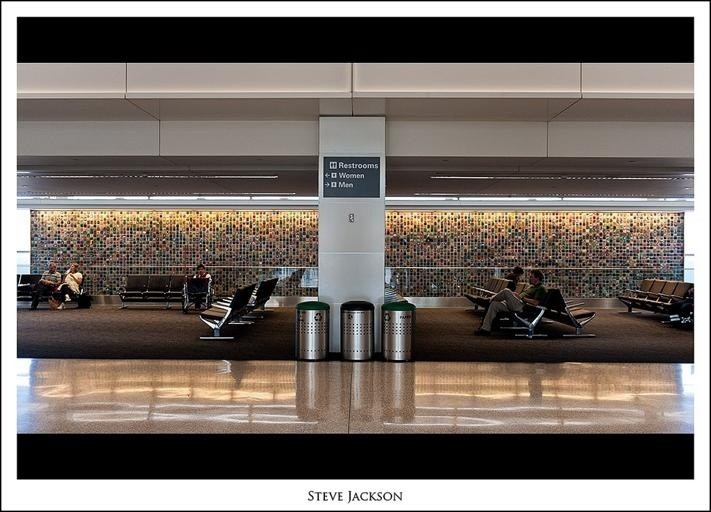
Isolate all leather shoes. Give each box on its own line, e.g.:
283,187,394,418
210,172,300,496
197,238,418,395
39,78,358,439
476,297,490,306
474,329,490,337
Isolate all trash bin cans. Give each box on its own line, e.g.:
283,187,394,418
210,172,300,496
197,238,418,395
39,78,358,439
296,301,330,361
341,301,375,361
382,301,416,362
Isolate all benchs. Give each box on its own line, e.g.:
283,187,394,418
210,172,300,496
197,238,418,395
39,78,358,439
119,273,203,314
616,277,695,326
199,277,279,340
17,271,86,309
462,275,598,338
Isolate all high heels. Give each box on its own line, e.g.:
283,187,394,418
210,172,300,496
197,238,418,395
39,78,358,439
57,294,71,310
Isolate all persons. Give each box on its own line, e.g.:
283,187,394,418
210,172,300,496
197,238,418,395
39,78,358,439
683,286,693,303
505,266,524,292
473,270,546,336
193,263,212,296
29,262,83,310
